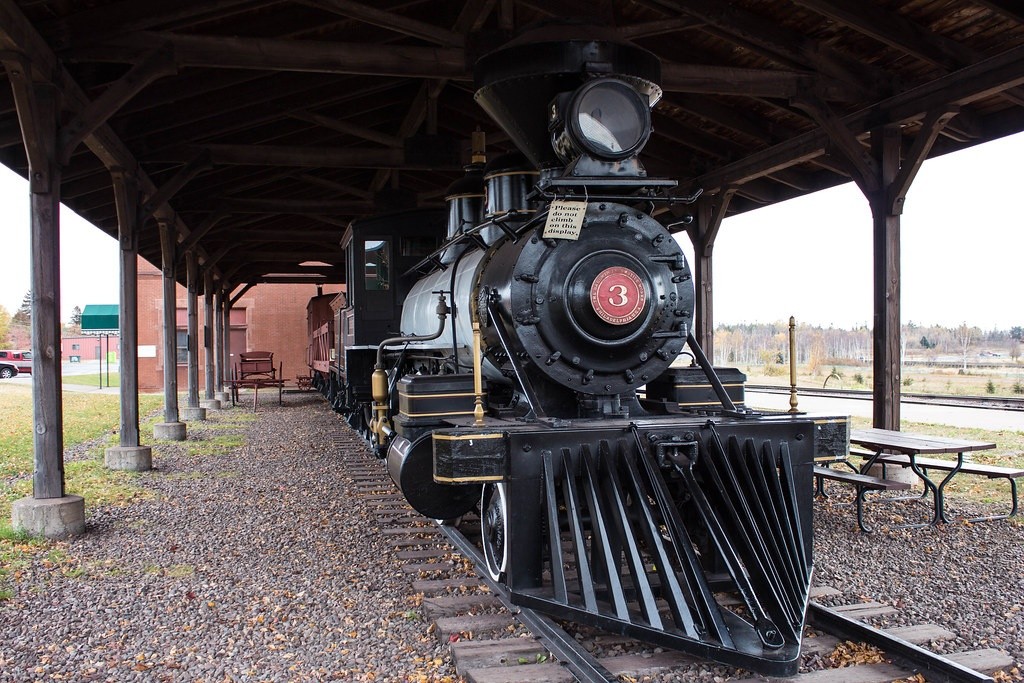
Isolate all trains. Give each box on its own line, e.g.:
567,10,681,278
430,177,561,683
306,78,815,678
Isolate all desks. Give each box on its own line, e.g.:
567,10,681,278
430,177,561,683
850,428,997,530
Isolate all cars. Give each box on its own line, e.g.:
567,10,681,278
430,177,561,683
0,363,19,378
0,350,32,375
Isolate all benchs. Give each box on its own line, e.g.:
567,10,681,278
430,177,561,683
863,454,1024,525
814,464,911,532
